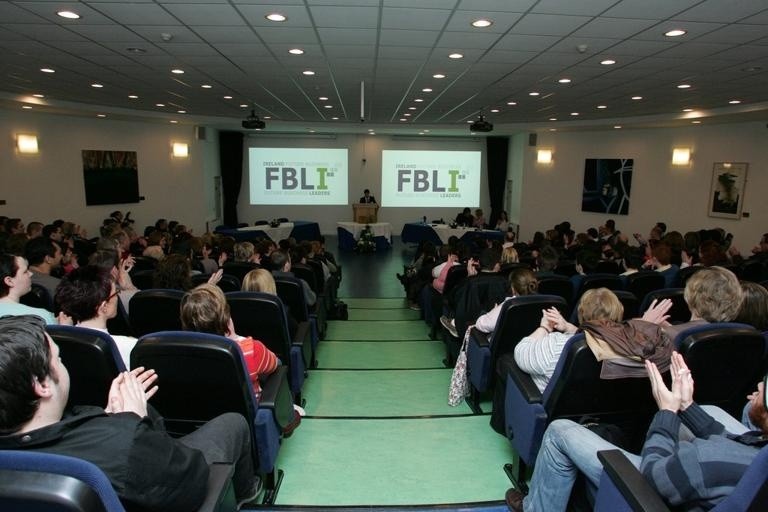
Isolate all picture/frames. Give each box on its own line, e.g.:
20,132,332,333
706,159,751,221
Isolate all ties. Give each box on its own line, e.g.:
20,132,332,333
367,197,369,203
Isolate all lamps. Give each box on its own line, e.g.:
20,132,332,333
171,68,183,76
13,133,40,155
449,52,460,61
601,59,618,68
264,12,288,25
559,76,570,83
287,46,305,55
171,141,193,162
39,67,55,75
664,28,688,38
535,146,555,168
670,145,691,166
92,82,103,89
55,8,92,27
675,82,693,90
729,99,738,108
470,17,495,32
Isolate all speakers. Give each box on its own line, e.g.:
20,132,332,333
195,126,205,140
527,133,536,146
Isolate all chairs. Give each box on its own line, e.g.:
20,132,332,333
427,264,466,341
0,448,130,512
641,288,689,327
430,219,445,225
593,447,673,512
625,268,663,292
313,252,342,303
236,223,249,229
497,325,676,501
482,222,496,229
221,256,265,288
188,270,240,304
671,321,713,404
553,260,578,280
576,275,622,293
121,254,160,284
441,274,508,370
128,331,286,511
253,219,270,227
532,278,576,302
464,296,569,416
215,224,237,231
127,286,197,334
41,323,136,408
276,217,289,223
291,265,331,338
237,290,306,422
270,270,318,382
306,259,336,311
20,282,56,315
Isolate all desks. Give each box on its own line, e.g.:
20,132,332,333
235,219,324,248
336,220,393,253
402,220,503,261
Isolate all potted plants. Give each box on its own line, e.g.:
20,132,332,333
267,217,280,229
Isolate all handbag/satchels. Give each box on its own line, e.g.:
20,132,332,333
447,325,477,407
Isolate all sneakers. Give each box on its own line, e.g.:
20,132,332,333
440,315,459,338
283,411,302,439
238,475,263,508
505,487,526,510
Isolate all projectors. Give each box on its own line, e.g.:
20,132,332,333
240,121,264,129
469,120,493,132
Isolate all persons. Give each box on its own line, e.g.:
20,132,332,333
358,188,378,203
505,352,768,512
447,235,465,251
420,248,463,320
471,208,487,226
529,219,768,274
428,242,482,339
464,229,538,273
285,245,331,307
1,314,278,507
23,236,63,295
456,207,474,227
513,287,624,395
242,269,308,411
496,210,509,230
632,267,743,349
52,211,214,295
400,223,443,267
268,249,329,341
709,173,738,212
208,229,270,288
180,269,301,438
440,251,499,370
0,216,46,257
54,267,140,371
273,237,334,255
448,268,544,407
0,253,55,326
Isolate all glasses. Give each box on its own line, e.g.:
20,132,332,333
96,284,123,310
